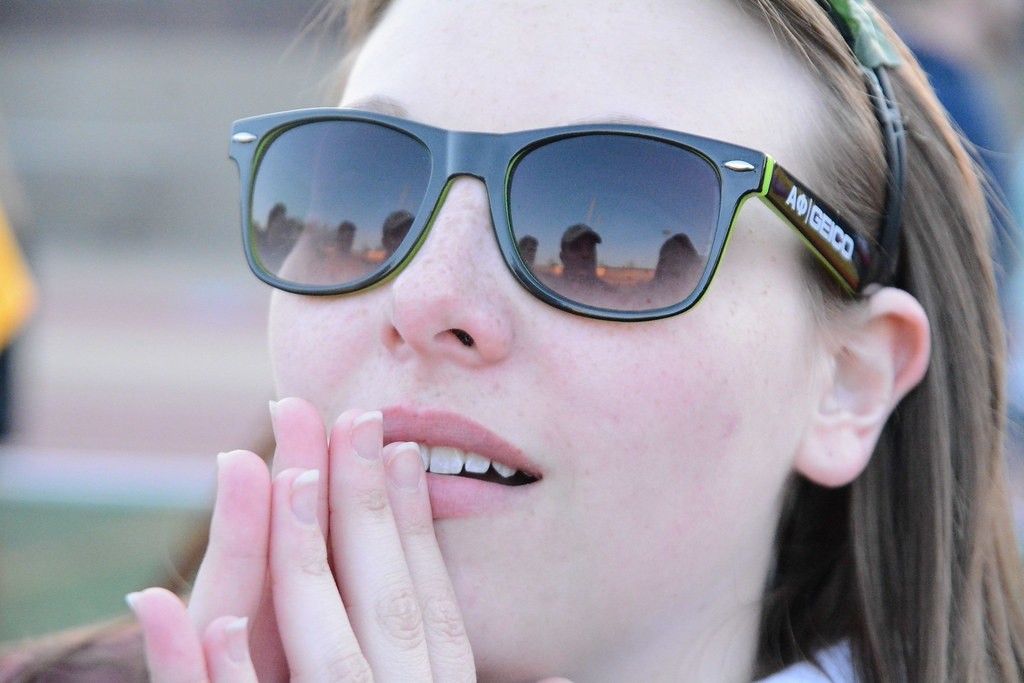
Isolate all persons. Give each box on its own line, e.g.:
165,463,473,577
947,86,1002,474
259,204,291,268
653,233,700,285
382,210,415,260
560,224,616,290
336,222,357,257
519,235,538,265
0,1,1024,683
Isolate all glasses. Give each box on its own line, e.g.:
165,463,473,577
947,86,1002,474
226,103,871,320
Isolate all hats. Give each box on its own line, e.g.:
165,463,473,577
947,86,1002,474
560,224,602,251
382,210,415,234
